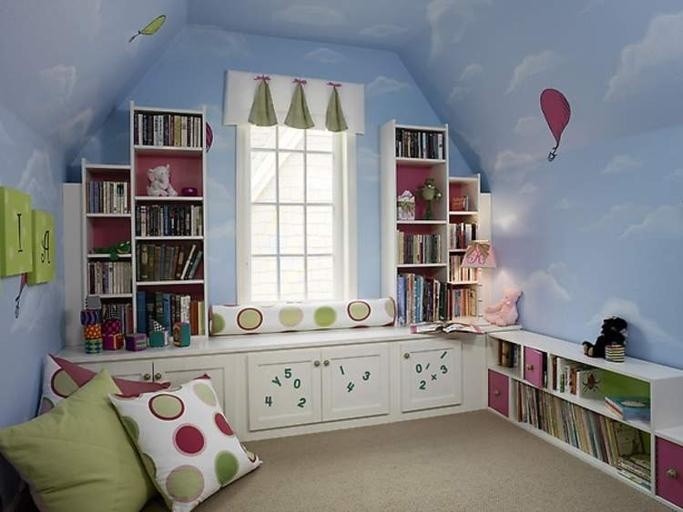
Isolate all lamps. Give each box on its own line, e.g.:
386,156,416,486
460,240,496,326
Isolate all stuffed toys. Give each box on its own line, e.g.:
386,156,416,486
482,285,523,327
581,314,629,358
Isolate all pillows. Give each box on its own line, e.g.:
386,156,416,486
49,354,171,398
1,367,158,512
4,352,80,512
108,372,263,512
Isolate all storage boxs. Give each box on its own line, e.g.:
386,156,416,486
397,200,416,220
80,310,124,354
125,331,165,350
173,322,190,347
86,295,102,310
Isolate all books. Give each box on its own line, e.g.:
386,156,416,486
495,340,650,489
82,112,205,337
394,125,477,327
408,320,483,335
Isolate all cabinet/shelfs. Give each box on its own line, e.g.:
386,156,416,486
75,351,249,443
248,340,401,441
81,157,137,345
430,172,479,324
401,329,521,422
380,118,450,328
130,101,208,343
485,330,683,512
